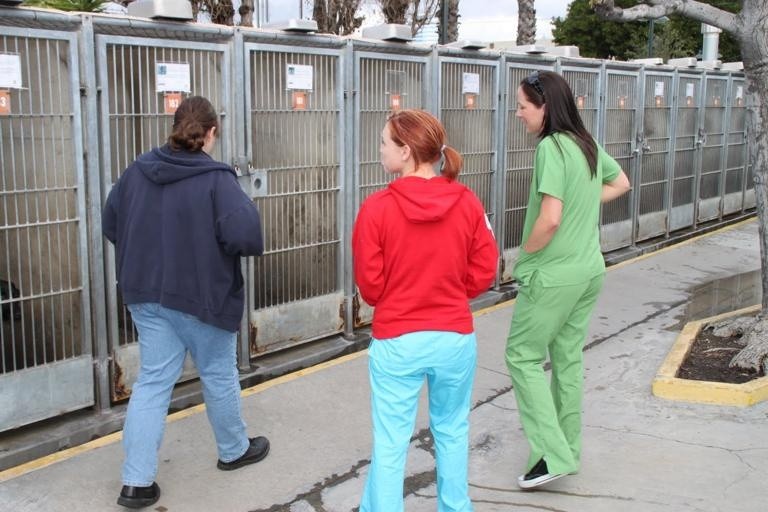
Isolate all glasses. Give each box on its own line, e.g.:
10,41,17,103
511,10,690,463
527,69,545,96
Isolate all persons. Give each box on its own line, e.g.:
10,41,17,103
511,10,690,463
506,72,630,490
351,108,499,511
102,97,269,508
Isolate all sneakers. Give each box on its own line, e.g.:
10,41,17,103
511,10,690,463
518,456,564,489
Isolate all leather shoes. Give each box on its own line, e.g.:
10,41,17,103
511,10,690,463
217,435,270,472
116,481,161,508
